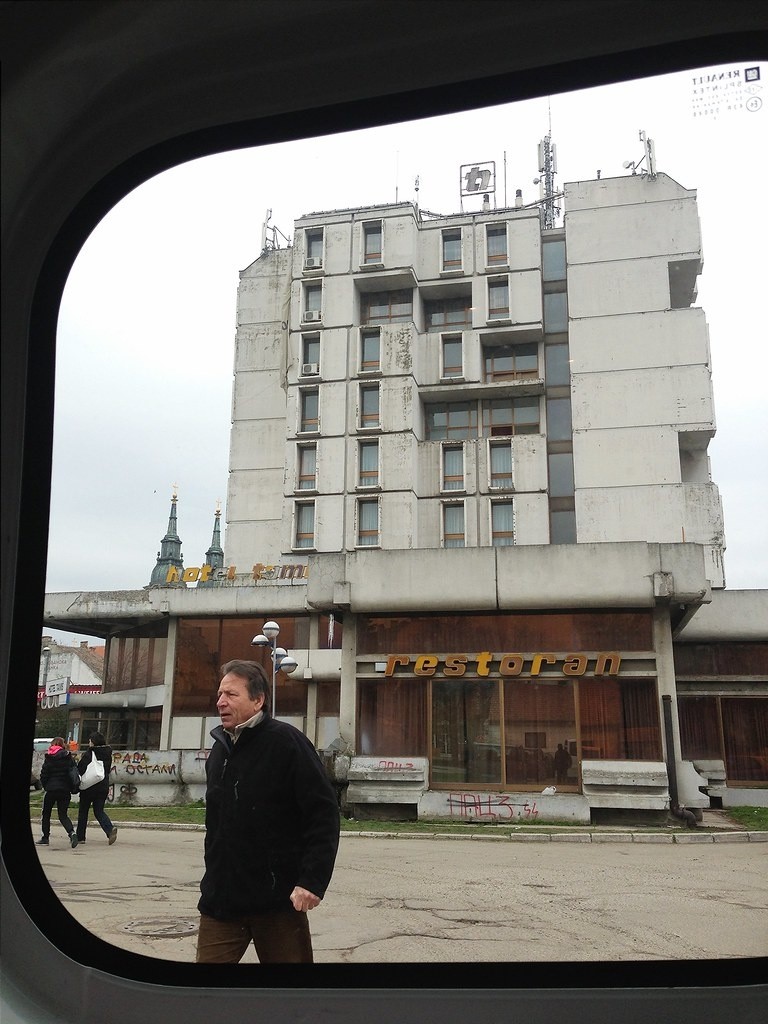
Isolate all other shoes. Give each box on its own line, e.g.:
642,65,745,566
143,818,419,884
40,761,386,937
77,841,85,844
109,826,118,845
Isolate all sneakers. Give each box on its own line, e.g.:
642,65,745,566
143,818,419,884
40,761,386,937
68,834,79,848
35,839,50,846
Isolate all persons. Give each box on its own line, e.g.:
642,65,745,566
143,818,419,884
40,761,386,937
35,737,79,848
75,731,117,845
554,743,573,783
192,660,342,967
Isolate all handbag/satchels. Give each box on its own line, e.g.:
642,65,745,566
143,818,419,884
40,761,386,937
79,751,106,790
67,756,82,792
